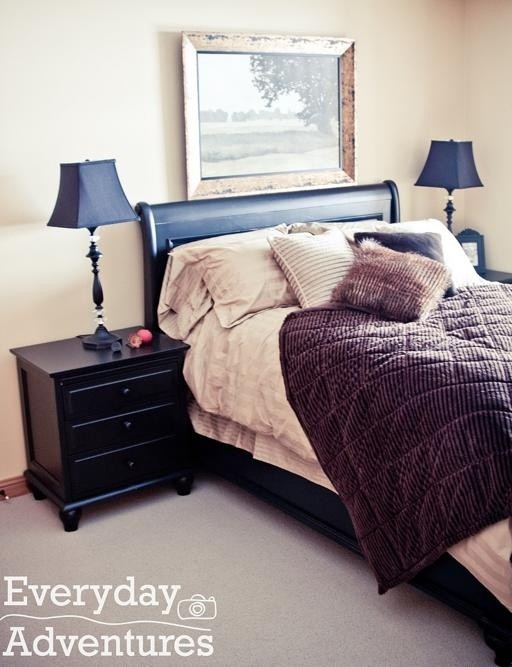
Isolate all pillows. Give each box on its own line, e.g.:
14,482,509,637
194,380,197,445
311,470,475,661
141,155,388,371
265,226,354,311
375,218,488,297
172,223,291,329
329,238,452,322
354,232,456,299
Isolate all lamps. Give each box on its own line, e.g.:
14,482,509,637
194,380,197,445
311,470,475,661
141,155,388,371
414,139,484,236
45,159,141,351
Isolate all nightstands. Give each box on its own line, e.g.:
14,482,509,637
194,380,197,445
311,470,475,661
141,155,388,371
9,325,190,531
481,269,512,284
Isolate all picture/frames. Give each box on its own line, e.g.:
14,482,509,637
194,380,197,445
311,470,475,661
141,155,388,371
455,229,486,278
181,32,355,202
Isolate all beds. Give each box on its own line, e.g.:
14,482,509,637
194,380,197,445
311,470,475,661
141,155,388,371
134,181,512,665
